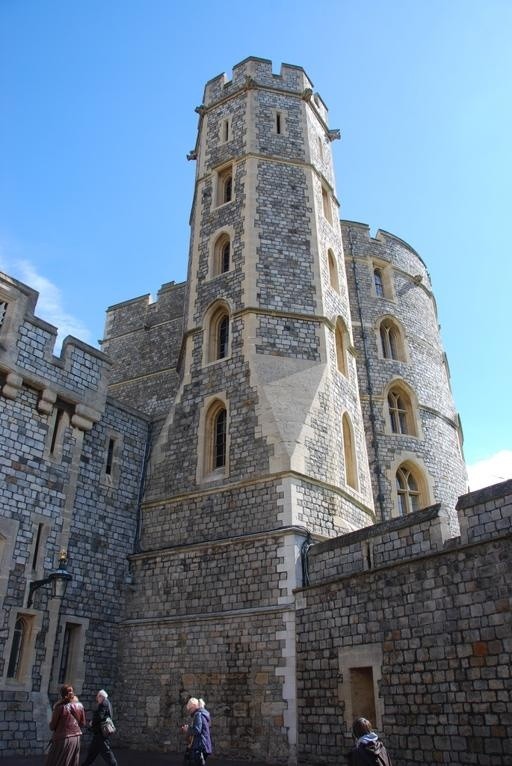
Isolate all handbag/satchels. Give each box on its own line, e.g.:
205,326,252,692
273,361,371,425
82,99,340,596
100,718,117,736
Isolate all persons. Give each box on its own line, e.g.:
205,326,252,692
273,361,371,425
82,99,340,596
182,697,212,766
44,683,79,766
82,690,117,766
349,718,391,766
50,685,85,766
